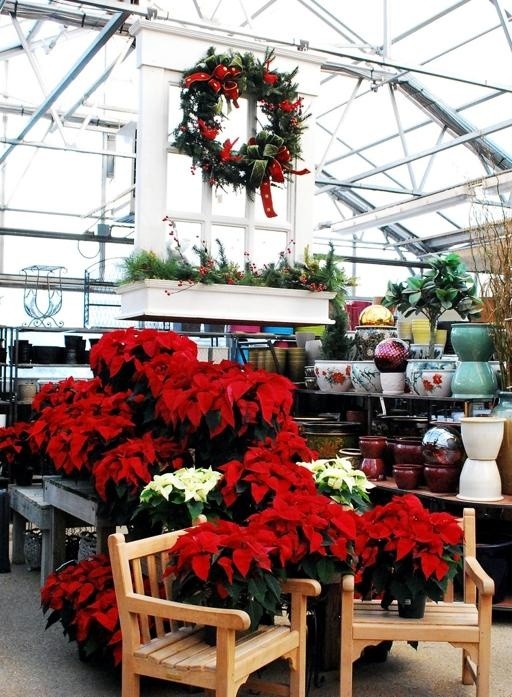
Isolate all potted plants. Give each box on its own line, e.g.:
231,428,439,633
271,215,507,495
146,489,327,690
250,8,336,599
380,253,485,395
466,150,512,496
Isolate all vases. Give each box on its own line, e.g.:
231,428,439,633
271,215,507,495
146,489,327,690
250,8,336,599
115,279,338,326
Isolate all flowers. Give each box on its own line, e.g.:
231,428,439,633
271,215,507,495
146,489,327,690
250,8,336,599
168,45,310,219
117,216,357,309
